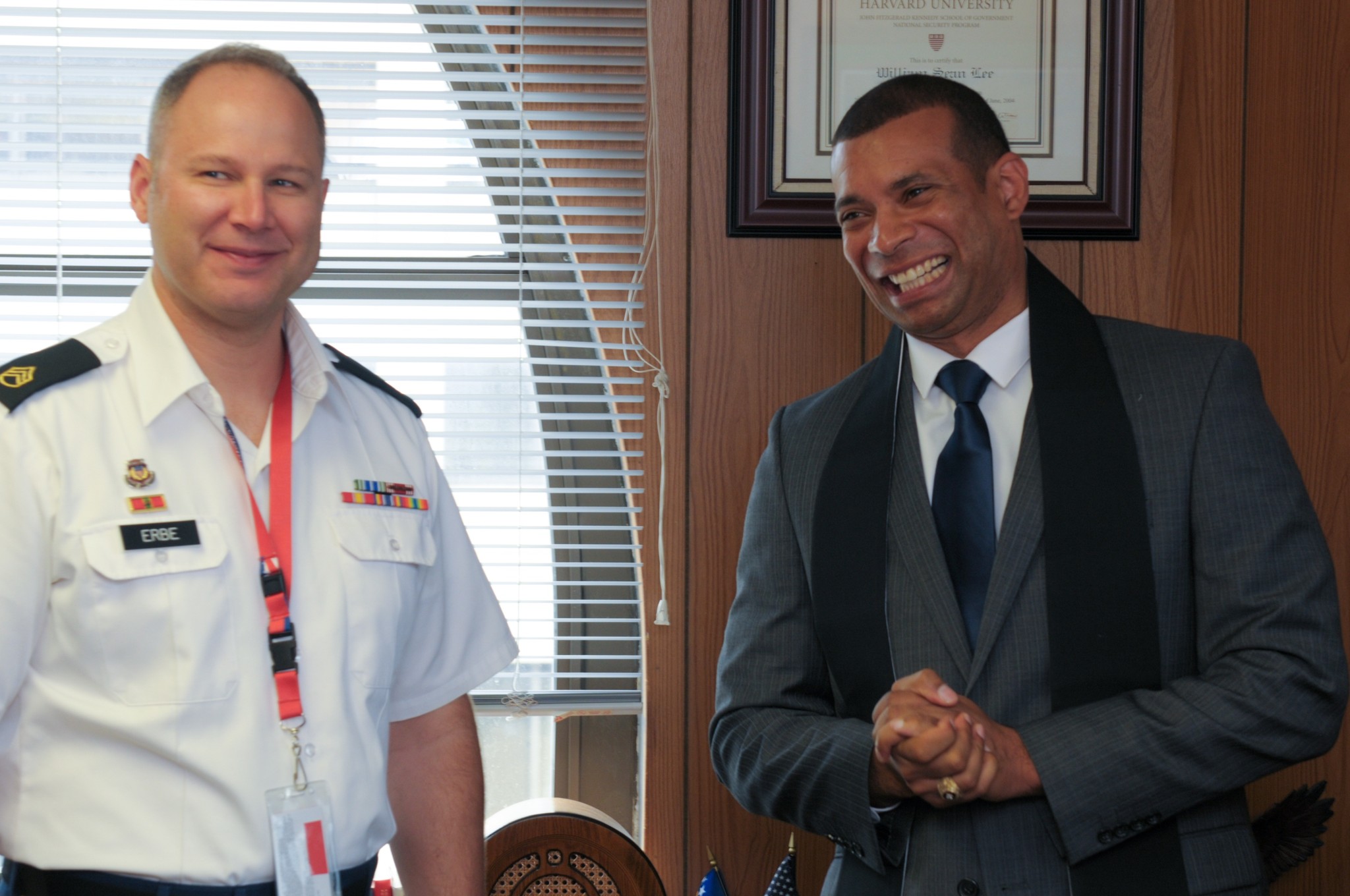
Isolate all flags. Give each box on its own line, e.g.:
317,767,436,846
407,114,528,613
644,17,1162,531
764,855,799,896
696,868,726,896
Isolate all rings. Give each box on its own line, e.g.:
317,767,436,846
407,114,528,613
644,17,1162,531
937,776,961,802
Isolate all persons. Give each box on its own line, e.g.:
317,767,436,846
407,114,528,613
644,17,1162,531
708,74,1350,896
0,45,519,896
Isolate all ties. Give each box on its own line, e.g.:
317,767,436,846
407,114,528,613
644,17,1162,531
930,359,995,658
